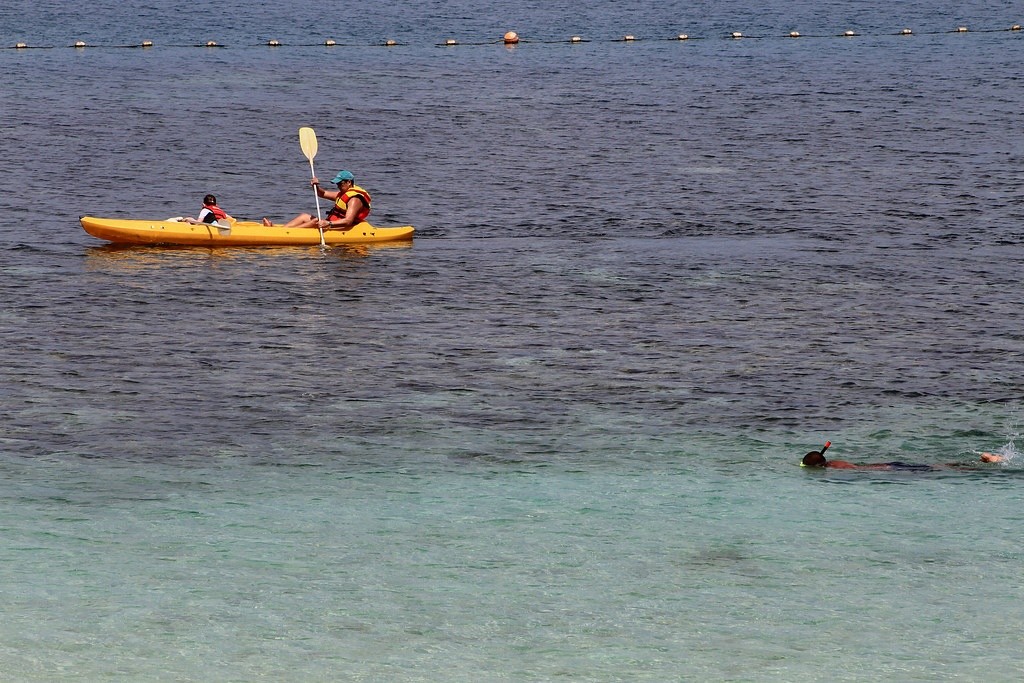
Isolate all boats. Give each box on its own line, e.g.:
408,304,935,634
76,213,415,243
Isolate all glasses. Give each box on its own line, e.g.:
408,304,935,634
336,182,347,185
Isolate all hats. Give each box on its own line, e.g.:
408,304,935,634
329,170,355,184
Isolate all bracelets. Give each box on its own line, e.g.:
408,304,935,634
329,222,332,225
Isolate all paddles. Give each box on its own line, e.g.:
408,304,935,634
178,217,232,236
299,127,326,247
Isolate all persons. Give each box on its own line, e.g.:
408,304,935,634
799,450,1003,472
181,194,235,226
263,170,371,231
